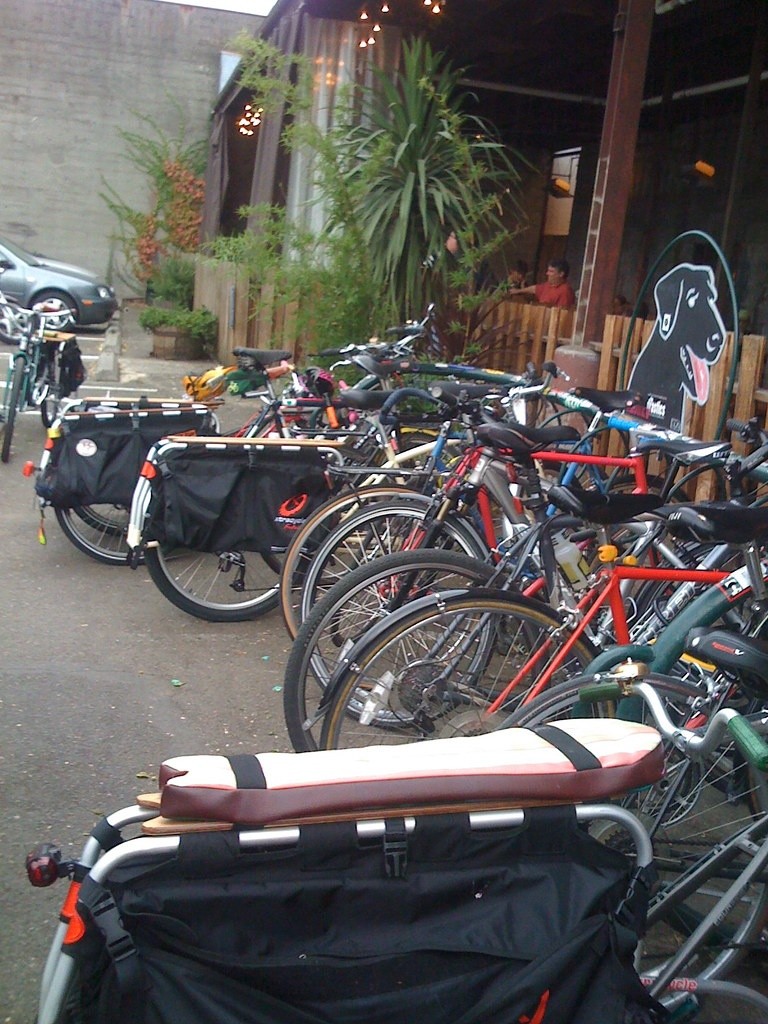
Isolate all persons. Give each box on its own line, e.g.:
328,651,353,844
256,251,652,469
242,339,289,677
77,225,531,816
492,261,528,295
614,295,649,319
509,258,574,306
422,221,509,359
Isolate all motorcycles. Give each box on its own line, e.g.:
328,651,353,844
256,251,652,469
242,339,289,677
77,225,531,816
0,280,34,344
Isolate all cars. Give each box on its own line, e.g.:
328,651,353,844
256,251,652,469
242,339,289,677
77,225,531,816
0,235,118,332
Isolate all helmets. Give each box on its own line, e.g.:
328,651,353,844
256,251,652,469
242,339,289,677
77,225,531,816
184,366,237,401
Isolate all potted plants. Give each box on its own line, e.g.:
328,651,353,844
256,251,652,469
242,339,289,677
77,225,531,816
137,305,219,361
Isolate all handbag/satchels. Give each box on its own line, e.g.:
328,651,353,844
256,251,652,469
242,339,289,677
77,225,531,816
58,808,672,1024
35,410,216,509
142,446,339,553
38,337,85,391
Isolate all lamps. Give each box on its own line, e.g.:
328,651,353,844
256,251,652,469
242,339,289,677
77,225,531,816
542,176,575,199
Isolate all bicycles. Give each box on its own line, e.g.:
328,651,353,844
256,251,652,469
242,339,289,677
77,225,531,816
128,367,568,620
38,322,454,572
273,379,768,752
0,295,77,463
29,633,768,1024
